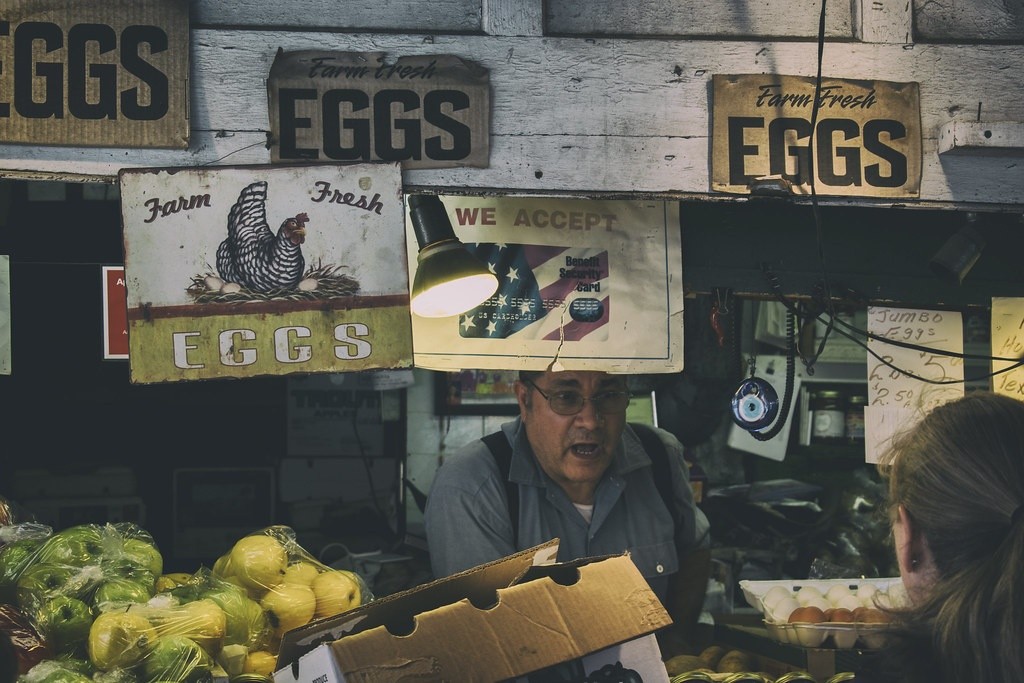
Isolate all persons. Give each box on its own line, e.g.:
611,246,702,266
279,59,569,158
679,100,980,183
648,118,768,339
851,392,1023,683
425,370,712,643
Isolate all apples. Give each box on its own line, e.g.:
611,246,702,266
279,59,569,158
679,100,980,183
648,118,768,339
0,522,361,683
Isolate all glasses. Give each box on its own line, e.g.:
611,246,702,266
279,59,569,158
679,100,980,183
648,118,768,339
520,371,635,417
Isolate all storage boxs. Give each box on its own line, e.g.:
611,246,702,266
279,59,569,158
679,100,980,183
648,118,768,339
270,536,678,683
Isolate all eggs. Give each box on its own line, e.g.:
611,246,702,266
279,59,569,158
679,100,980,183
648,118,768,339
764,583,906,623
220,282,242,294
298,278,318,292
204,276,224,291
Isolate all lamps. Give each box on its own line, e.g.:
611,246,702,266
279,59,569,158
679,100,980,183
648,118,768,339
406,192,498,319
